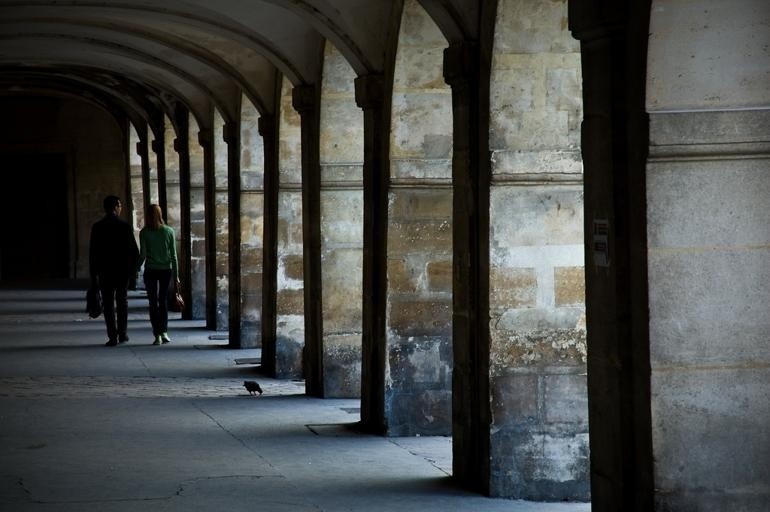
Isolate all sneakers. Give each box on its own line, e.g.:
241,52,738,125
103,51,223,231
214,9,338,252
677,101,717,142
106,336,128,346
153,332,170,344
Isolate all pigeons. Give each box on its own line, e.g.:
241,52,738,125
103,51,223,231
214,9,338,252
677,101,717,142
242,380,263,396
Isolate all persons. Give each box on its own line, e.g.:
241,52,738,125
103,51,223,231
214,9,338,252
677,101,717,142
89,195,139,346
136,204,180,345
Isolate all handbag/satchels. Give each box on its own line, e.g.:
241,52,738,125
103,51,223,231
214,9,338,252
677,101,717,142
168,292,185,313
80,278,104,318
